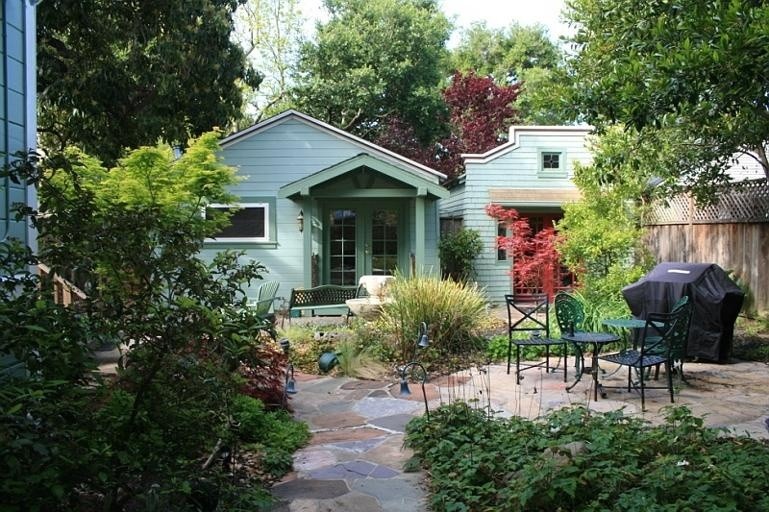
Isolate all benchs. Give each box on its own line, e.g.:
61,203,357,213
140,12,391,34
279,282,362,331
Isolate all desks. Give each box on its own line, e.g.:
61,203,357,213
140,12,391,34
601,319,677,399
560,332,623,402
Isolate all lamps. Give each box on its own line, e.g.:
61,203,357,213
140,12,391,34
297,209,304,233
397,361,433,430
282,364,297,404
411,321,432,379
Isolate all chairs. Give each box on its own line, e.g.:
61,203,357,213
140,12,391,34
504,292,568,387
591,304,682,412
630,301,695,393
547,294,606,381
243,277,283,339
641,295,692,381
